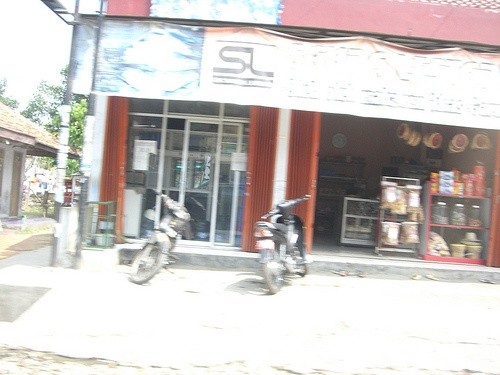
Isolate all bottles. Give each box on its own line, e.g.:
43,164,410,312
433,202,449,225
450,203,468,226
467,205,483,226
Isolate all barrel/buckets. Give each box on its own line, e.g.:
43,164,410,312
381,181,423,215
381,221,420,246
449,244,483,260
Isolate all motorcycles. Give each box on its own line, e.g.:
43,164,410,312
125,185,195,285
253,193,312,295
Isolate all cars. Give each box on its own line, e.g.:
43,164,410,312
24,166,81,207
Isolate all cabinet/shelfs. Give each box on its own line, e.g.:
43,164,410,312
89,201,116,247
314,159,491,265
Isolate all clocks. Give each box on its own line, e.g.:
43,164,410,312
332,134,347,148
